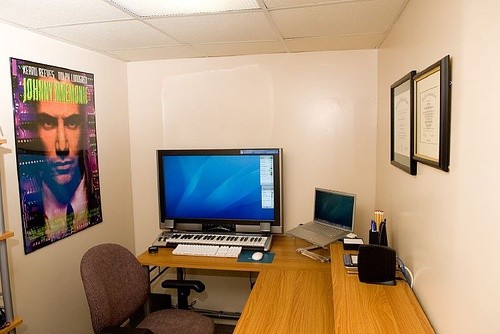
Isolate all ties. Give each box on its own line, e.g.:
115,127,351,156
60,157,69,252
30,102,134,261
66,204,74,235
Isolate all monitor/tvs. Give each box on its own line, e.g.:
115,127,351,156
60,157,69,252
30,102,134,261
156,149,282,236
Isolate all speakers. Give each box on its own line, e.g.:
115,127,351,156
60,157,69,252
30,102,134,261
357,243,397,286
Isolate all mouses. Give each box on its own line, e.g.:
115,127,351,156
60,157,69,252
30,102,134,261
252,251,263,261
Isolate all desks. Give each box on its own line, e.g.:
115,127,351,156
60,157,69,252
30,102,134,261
137,234,436,334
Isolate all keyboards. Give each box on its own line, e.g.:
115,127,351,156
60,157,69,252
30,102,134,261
171,244,243,259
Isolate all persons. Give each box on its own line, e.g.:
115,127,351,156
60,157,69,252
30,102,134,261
23,63,102,250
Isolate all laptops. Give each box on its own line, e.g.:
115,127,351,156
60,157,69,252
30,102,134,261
283,187,357,247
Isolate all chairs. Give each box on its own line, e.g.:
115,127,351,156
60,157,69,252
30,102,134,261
80,243,215,334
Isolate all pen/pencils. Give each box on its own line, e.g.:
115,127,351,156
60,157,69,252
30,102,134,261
375,210,383,232
